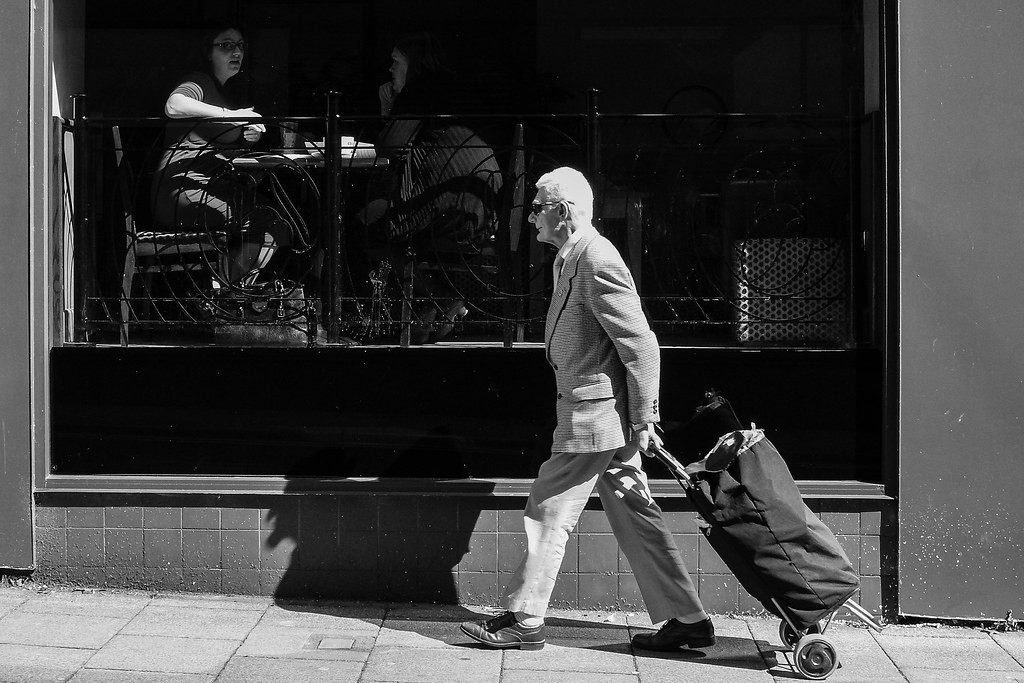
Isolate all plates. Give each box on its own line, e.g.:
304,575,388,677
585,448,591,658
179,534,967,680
312,152,357,158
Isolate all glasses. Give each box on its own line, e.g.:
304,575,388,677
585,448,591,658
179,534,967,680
530,200,574,216
212,41,246,51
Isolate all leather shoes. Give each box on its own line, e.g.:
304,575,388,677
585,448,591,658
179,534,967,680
460,612,546,650
632,617,716,651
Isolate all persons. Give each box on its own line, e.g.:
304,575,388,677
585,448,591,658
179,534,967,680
460,167,715,650
379,34,469,345
152,26,267,331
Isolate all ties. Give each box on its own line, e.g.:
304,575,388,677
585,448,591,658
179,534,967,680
553,253,564,293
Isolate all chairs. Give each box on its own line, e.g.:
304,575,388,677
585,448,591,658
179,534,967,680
401,123,526,348
113,125,229,347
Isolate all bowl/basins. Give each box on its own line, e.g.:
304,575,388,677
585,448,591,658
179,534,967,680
305,142,323,154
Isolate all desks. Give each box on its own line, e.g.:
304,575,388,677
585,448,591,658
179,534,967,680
232,158,391,347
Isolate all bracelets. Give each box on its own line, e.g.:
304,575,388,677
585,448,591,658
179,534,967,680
382,108,392,112
630,423,648,433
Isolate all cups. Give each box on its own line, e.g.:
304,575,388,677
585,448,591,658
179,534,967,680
280,122,299,148
